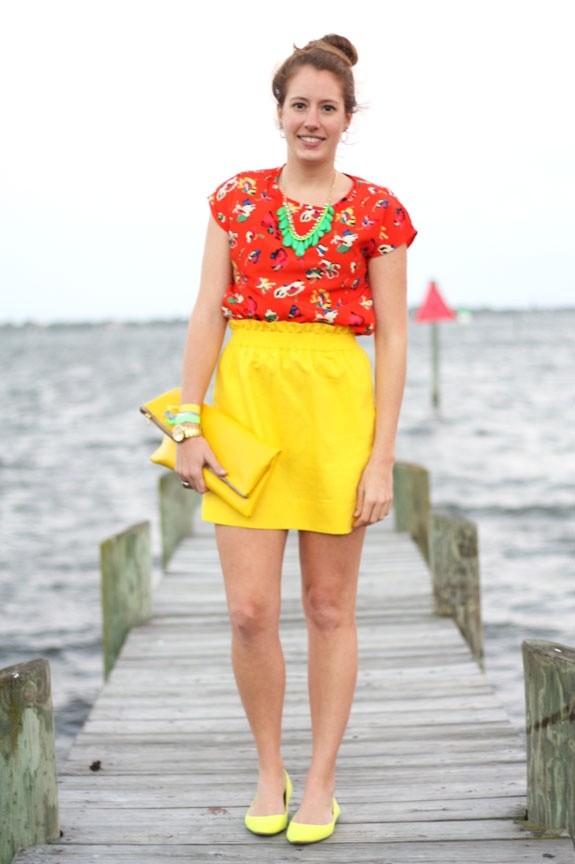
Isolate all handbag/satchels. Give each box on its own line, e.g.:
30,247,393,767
136,387,279,515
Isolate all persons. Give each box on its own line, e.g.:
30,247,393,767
174,33,418,844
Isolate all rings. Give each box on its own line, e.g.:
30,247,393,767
181,481,190,488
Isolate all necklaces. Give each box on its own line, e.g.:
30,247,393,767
277,169,338,257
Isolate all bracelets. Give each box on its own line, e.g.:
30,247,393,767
167,403,201,424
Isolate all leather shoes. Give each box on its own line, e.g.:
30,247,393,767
287,796,340,842
244,770,292,835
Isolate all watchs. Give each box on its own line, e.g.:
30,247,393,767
172,424,202,443
